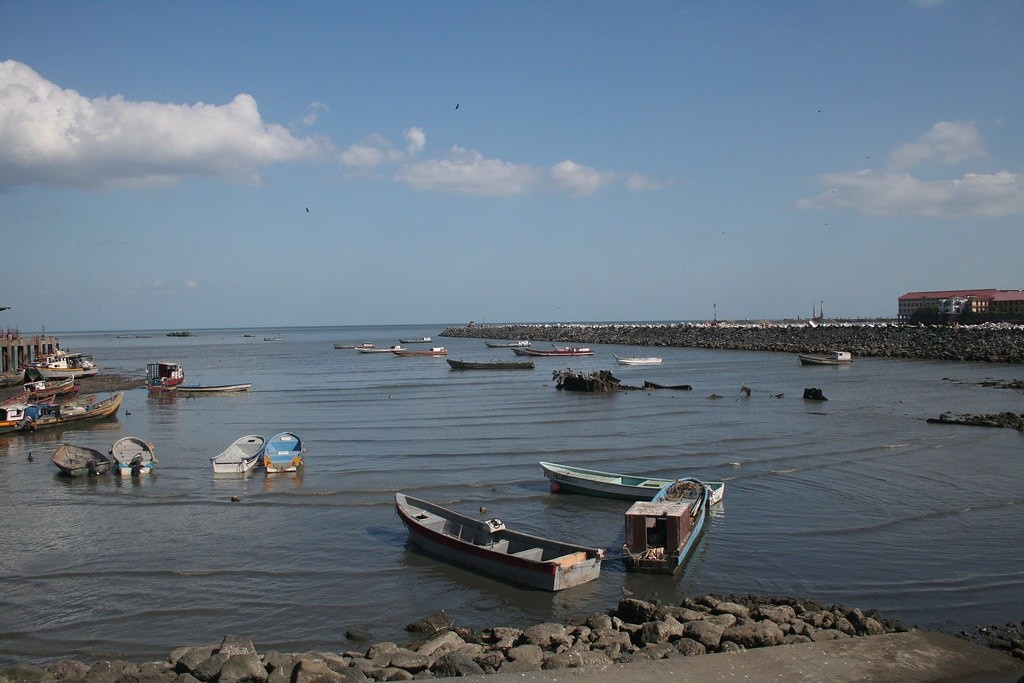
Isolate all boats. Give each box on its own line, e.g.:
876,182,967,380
263,432,304,472
539,460,724,507
799,350,852,367
210,434,265,472
244,334,285,341
358,344,407,353
51,442,110,478
399,337,432,343
176,384,252,391
622,477,708,574
395,493,605,591
444,358,535,369
117,331,191,338
485,340,531,348
333,342,375,349
512,344,595,357
145,362,184,388
393,347,448,356
0,348,124,434
111,437,156,475
613,354,662,365
